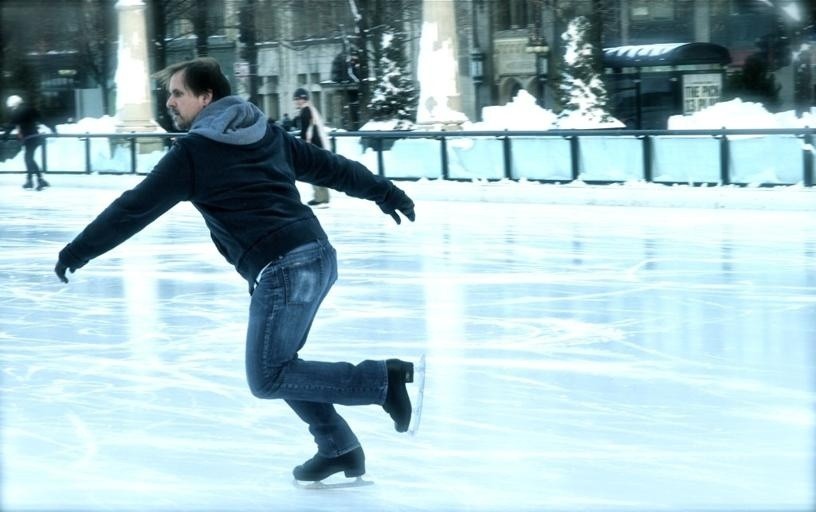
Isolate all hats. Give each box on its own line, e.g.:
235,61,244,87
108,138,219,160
293,88,309,100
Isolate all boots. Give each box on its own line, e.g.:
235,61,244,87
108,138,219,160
22,179,49,191
293,447,365,481
308,199,328,206
383,359,414,432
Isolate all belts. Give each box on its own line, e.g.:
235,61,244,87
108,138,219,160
253,261,272,289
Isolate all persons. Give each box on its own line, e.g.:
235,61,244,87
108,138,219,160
54,58,414,482
0,95,59,192
291,88,331,206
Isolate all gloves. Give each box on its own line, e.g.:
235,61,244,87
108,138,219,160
390,193,415,225
55,260,76,283
